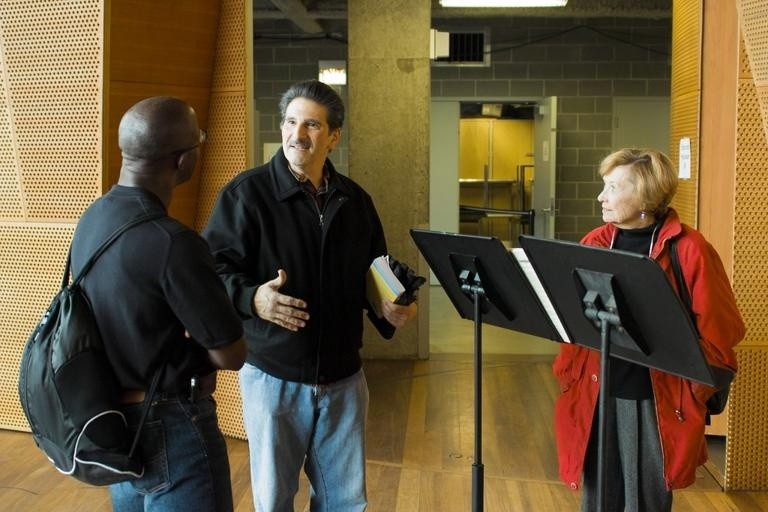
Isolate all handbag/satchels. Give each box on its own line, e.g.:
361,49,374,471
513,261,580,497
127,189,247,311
705,362,734,414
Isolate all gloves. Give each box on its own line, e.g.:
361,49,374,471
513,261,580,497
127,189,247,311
366,260,426,340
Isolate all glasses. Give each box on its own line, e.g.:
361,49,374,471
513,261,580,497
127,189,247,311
172,129,208,153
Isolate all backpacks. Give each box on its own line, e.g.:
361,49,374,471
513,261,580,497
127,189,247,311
17,206,163,486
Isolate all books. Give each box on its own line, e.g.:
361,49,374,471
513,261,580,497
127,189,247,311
364,253,406,321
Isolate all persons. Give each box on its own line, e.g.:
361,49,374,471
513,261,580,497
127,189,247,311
551,147,747,512
71,96,247,512
201,79,417,512
